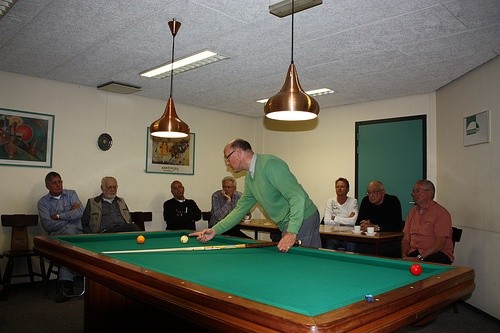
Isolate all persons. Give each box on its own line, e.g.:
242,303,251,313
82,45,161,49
323,177,358,252
82,176,140,233
355,180,402,259
187,137,322,253
164,180,201,231
208,175,253,238
38,171,85,303
401,180,455,265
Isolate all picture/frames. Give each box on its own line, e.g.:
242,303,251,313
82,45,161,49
0,108,55,168
146,127,195,175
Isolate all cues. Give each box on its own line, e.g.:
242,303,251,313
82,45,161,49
101,239,302,255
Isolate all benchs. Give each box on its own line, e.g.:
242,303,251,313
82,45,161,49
0,212,153,299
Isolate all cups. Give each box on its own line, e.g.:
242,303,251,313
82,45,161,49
367,227,374,233
354,226,361,231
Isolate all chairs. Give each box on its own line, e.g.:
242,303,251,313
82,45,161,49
452,227,462,313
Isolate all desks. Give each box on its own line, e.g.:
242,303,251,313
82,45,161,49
235,218,403,256
31,229,476,333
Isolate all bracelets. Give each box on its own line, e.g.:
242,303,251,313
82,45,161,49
377,224,380,232
56,213,60,219
416,255,424,261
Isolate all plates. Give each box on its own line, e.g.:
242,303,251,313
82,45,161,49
351,230,363,234
364,232,377,236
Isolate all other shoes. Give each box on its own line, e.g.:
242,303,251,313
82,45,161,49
54,284,74,303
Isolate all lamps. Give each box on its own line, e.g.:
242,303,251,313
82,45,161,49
96,81,141,95
264,0,320,121
150,18,190,138
269,0,323,18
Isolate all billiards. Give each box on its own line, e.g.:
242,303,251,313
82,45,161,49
180,235,188,244
137,235,145,244
410,263,423,276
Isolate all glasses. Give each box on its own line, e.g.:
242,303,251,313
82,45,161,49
223,150,236,163
102,185,118,189
365,188,385,197
46,180,63,185
411,187,432,194
